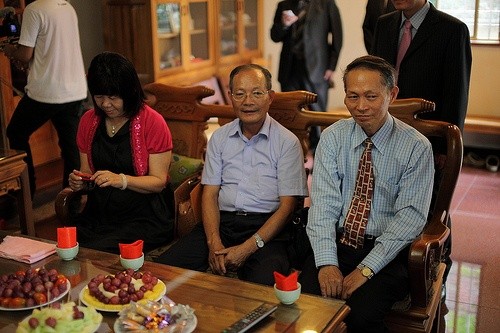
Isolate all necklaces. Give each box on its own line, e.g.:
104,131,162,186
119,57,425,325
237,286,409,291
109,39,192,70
111,118,127,134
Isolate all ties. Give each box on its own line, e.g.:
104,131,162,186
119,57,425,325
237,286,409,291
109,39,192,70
339,139,375,250
395,21,412,85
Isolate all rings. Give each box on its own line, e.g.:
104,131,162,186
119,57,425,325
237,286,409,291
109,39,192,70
106,178,108,181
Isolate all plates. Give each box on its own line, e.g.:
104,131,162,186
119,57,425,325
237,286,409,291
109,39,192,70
16,306,100,333
0,278,71,310
79,274,167,312
114,306,198,333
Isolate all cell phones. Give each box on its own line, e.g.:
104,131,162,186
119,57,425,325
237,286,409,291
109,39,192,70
282,9,295,17
75,172,95,181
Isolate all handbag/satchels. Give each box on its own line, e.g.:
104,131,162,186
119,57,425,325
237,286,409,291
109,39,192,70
173,173,202,239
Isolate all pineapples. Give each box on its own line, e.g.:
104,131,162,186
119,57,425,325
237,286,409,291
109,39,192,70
83,281,165,311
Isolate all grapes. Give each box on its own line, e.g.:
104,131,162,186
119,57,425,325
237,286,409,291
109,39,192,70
88,268,158,304
0,266,67,308
29,302,84,328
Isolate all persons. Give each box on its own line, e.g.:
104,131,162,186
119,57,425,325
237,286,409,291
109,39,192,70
4,0,89,204
69,52,176,263
154,64,310,287
306,55,435,333
362,0,473,305
270,0,343,148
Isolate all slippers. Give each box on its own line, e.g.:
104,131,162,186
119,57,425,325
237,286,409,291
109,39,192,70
486,154,500,172
464,151,484,167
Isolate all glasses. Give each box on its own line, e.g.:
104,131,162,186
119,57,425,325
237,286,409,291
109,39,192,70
232,88,268,102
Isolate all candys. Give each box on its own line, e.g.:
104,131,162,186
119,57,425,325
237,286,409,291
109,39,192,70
118,299,187,333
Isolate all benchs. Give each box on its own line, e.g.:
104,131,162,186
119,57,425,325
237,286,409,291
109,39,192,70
54,83,464,333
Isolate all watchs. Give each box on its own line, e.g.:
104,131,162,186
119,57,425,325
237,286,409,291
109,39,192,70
357,264,373,279
253,233,265,248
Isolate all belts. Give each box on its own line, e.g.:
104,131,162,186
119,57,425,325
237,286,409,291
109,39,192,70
220,211,273,217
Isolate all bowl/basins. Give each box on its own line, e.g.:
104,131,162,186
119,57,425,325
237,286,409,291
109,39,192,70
119,252,144,272
55,242,79,260
274,282,301,305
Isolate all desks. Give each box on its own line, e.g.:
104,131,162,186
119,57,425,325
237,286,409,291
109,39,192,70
0,229,351,333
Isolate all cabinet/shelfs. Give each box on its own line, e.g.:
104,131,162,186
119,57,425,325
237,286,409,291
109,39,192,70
101,0,265,105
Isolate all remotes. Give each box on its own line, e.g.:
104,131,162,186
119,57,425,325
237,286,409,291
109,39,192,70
220,303,278,333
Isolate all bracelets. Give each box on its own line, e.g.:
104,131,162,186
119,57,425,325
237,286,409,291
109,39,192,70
118,173,128,190
13,44,17,57
